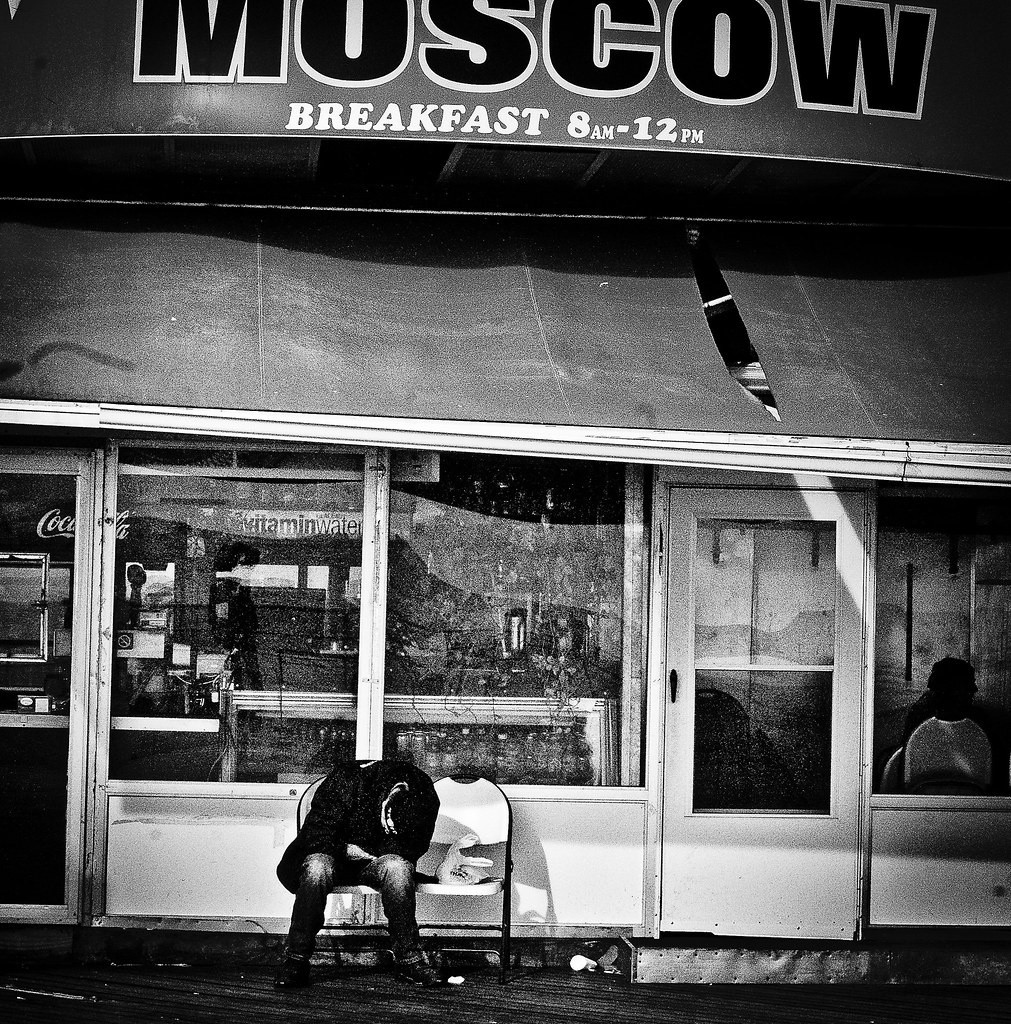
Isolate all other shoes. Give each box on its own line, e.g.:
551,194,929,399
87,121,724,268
398,962,443,987
274,958,310,989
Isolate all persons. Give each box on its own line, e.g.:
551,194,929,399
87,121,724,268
273,760,442,991
210,583,263,732
903,657,989,745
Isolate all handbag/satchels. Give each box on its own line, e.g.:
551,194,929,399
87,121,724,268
437,834,493,885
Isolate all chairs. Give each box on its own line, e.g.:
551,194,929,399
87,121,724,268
296,768,513,986
882,714,993,798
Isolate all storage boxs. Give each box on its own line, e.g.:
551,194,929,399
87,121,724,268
18,692,53,714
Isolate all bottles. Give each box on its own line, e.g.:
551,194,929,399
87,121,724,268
239,715,589,780
